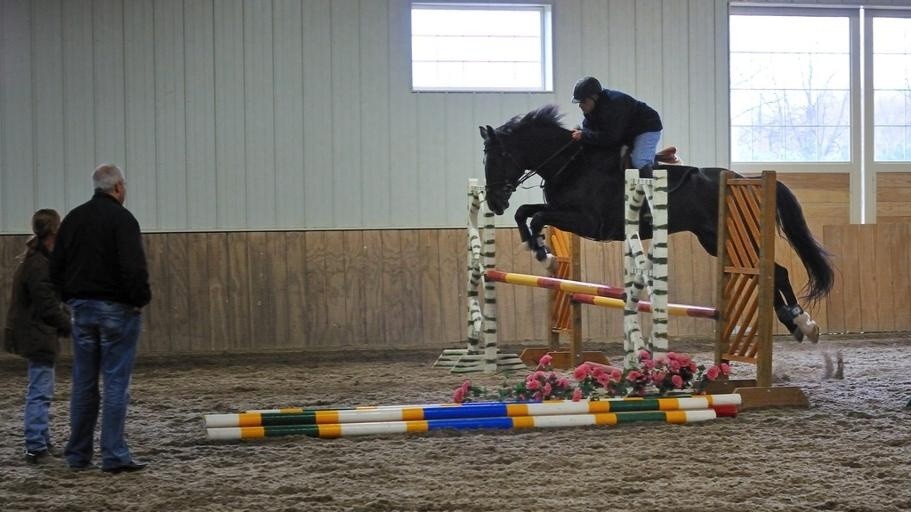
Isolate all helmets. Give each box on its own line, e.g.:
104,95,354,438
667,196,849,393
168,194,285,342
572,77,602,103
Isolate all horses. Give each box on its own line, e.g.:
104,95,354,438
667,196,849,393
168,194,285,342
479,104,838,344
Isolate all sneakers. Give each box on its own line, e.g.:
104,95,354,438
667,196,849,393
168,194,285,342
25,444,64,464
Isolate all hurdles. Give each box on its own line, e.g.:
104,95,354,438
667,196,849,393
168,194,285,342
433,170,814,412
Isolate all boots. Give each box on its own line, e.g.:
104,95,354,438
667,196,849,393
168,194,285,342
639,163,653,221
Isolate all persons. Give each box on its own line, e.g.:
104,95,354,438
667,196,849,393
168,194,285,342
50,163,152,473
0,208,74,465
570,77,663,227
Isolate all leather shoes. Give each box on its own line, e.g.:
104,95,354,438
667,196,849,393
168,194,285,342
80,459,148,474
